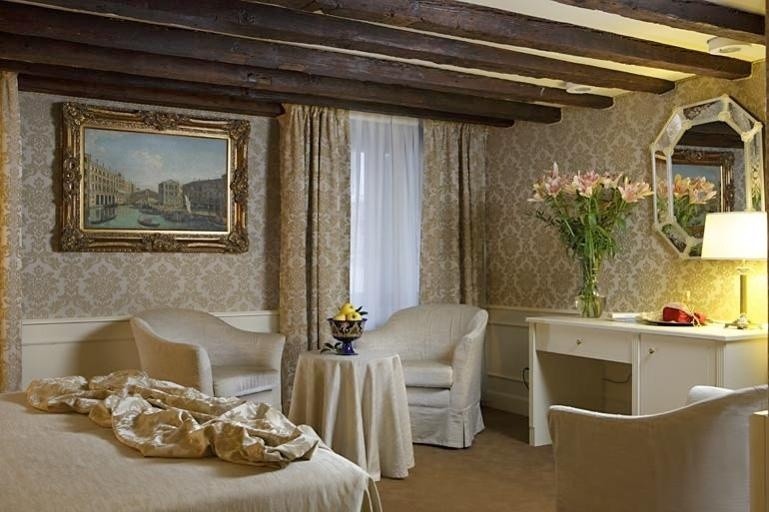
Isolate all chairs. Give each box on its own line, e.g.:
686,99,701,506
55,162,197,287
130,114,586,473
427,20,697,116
128,307,287,415
350,303,490,449
545,385,769,512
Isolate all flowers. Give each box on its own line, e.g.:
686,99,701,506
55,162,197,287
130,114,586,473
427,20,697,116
656,172,717,234
527,160,655,318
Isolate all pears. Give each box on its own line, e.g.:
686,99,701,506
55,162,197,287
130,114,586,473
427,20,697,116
333,303,361,320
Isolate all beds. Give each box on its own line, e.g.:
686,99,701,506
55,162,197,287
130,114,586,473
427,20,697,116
0,368,385,512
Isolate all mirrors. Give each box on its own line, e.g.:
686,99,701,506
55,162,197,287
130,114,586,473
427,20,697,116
648,93,765,261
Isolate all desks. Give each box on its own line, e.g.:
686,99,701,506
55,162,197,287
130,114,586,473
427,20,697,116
286,348,416,478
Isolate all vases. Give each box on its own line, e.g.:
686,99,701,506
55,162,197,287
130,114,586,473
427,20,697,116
575,275,607,318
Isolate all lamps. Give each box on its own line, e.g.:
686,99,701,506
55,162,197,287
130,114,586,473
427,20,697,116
701,210,768,331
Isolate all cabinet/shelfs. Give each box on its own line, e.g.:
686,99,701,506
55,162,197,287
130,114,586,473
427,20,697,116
525,314,769,447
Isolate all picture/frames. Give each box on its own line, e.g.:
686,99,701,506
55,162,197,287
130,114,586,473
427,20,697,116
57,100,251,255
